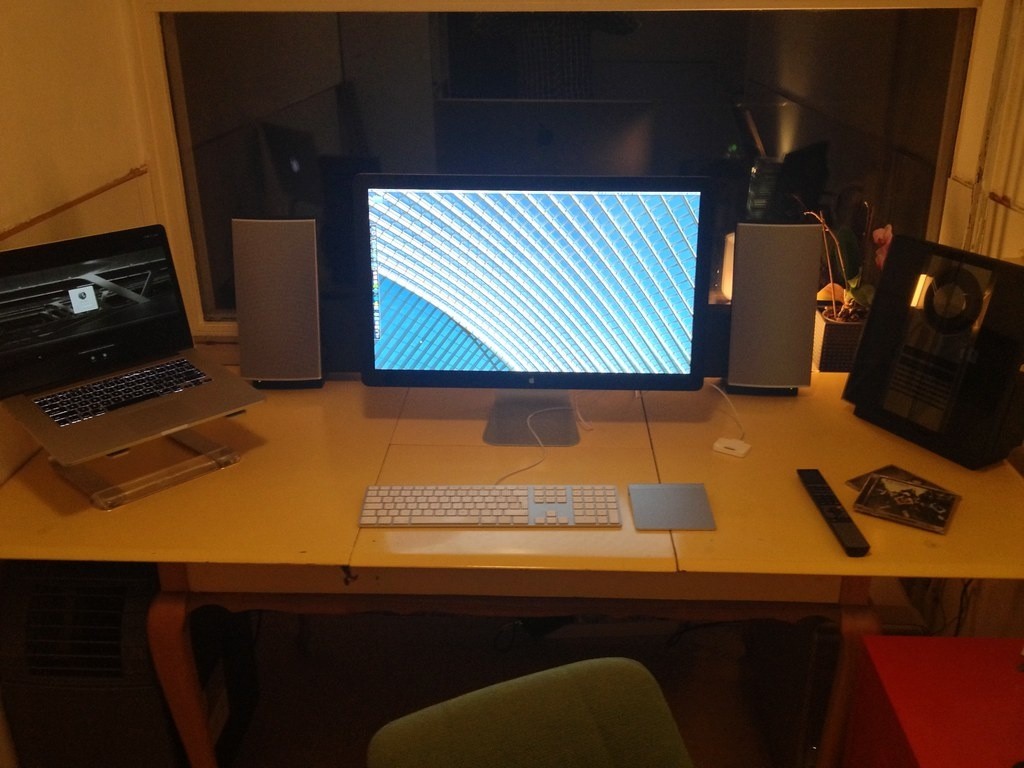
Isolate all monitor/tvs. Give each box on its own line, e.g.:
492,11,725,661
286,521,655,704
354,173,715,447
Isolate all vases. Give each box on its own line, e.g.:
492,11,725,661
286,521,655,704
814,305,866,372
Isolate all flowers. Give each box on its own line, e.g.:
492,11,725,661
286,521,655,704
816,222,892,316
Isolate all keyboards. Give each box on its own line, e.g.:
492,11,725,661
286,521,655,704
358,485,622,529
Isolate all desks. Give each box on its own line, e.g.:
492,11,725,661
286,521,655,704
4,365,1024,768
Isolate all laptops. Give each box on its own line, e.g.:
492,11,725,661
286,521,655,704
1,224,266,466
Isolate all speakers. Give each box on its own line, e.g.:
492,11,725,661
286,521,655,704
725,221,824,396
233,209,330,389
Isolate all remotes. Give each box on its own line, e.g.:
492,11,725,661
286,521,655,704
796,469,871,558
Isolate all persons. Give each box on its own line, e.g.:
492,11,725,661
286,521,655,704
471,11,640,100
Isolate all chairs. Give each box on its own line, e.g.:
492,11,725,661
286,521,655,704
366,658,694,768
